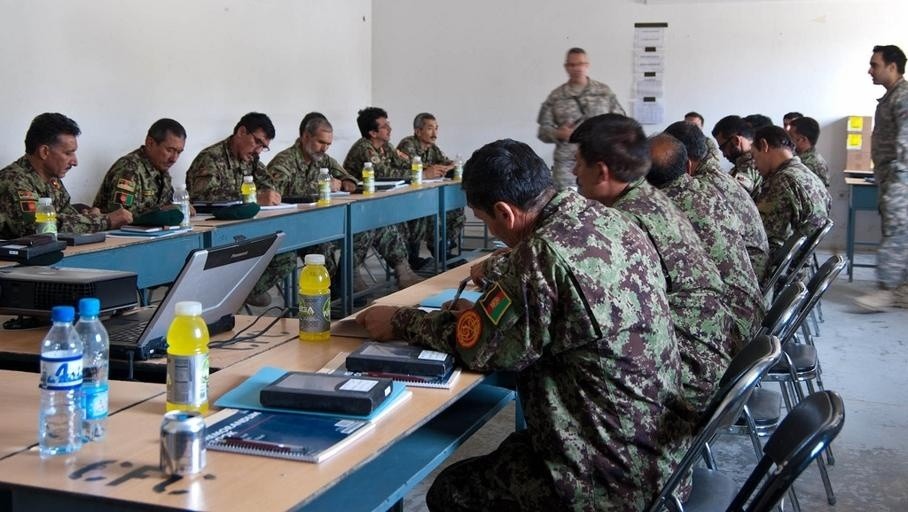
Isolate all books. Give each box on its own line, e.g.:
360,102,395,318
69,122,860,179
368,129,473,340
202,408,374,465
317,351,462,390
358,180,406,186
118,222,181,234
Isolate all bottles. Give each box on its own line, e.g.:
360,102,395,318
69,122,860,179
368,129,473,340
241,176,257,205
173,183,190,227
35,198,58,241
68,298,109,443
453,154,463,180
166,301,209,417
318,168,331,204
411,156,423,187
39,306,85,457
362,162,375,196
298,254,331,341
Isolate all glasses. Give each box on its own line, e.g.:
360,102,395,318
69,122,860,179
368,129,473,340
251,133,270,153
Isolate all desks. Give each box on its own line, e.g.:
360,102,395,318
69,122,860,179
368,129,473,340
844,177,879,283
188,199,357,319
0,226,216,308
327,252,494,339
394,178,488,279
0,369,167,458
292,182,447,317
0,336,529,512
0,306,299,370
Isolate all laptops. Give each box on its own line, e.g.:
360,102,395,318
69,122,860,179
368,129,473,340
102,231,286,361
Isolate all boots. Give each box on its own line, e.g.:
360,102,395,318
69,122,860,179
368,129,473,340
894,286,907,310
397,258,425,289
409,242,433,271
247,293,271,306
854,290,894,312
354,276,367,291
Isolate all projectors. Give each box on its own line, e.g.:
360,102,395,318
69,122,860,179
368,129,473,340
0,265,138,329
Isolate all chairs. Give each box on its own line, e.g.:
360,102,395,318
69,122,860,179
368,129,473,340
726,390,845,512
760,234,814,395
775,217,834,345
762,253,847,465
648,335,782,512
690,281,836,512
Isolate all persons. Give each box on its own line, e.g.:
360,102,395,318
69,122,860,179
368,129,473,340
92,118,198,219
358,138,693,512
0,112,135,237
647,111,834,345
342,106,427,272
299,112,429,289
537,47,626,191
469,110,745,427
272,117,370,309
186,111,298,307
854,43,908,314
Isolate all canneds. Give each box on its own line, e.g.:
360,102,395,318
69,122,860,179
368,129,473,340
159,409,207,478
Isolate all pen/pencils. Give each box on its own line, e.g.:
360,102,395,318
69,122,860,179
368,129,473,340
447,280,467,311
217,441,293,454
120,204,124,208
330,173,333,178
361,371,429,382
272,185,276,191
223,436,304,450
